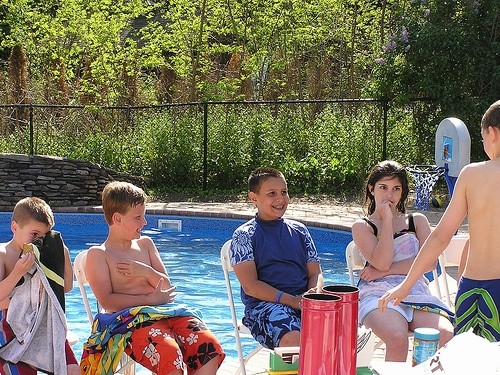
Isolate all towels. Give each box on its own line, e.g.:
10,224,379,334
356,232,456,327
0,230,67,375
80,303,203,375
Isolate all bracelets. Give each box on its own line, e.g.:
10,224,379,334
276,291,284,304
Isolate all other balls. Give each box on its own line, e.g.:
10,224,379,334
432,194,445,208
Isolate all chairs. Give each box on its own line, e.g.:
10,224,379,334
73,239,451,375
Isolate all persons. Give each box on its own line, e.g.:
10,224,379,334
379,100,500,344
0,196,81,375
85,181,225,375
352,161,453,362
457,238,470,286
229,168,323,350
